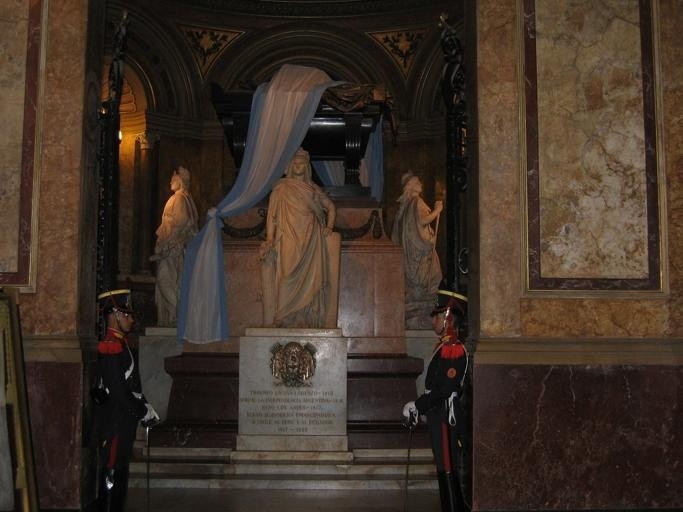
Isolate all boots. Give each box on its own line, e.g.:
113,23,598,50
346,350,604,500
437,470,465,512
98,470,130,512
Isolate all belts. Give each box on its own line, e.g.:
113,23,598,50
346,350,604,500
424,389,460,397
127,391,143,400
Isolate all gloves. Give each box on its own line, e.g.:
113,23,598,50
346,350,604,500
139,403,161,422
402,401,417,417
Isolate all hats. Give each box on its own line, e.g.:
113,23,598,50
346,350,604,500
98,288,133,316
429,289,469,318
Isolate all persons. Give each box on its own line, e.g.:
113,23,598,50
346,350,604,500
149,164,200,326
392,171,443,329
259,147,336,327
403,290,472,512
90,287,160,511
